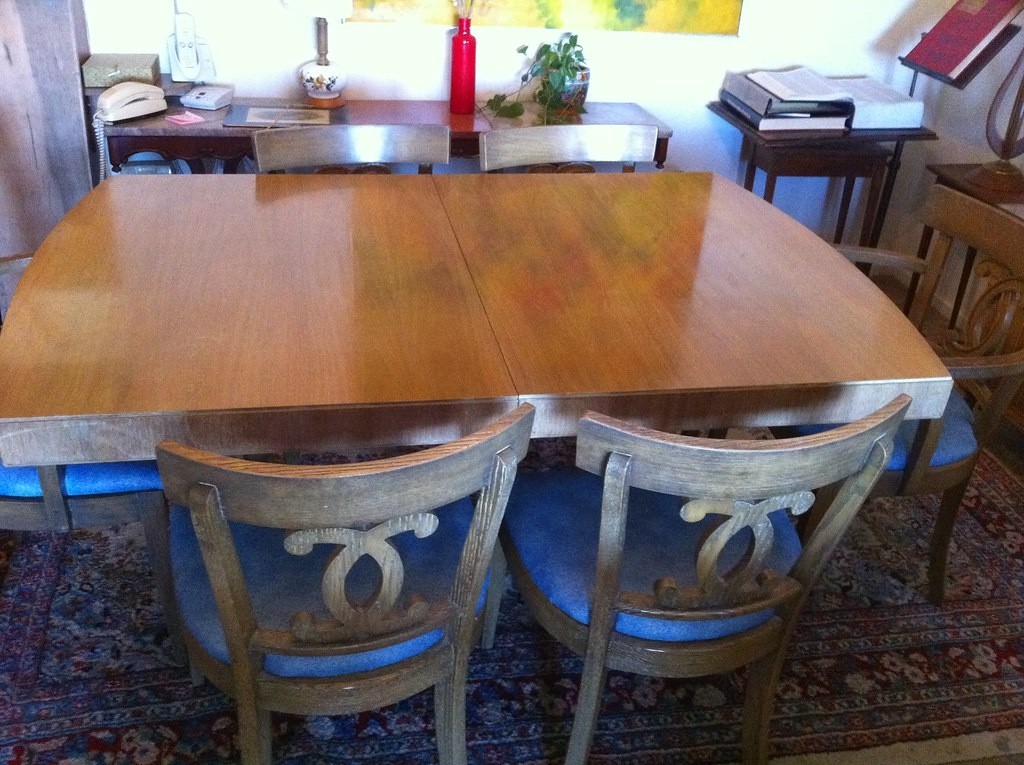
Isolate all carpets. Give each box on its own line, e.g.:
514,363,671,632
0,445,1024,765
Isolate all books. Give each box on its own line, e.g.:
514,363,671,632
904,0,1024,82
720,66,924,133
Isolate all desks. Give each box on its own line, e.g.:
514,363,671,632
905,164,1023,330
103,99,674,175
707,100,940,276
0,174,954,471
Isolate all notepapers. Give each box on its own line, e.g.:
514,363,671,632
164,111,205,127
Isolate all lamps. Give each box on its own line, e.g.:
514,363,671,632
282,0,355,108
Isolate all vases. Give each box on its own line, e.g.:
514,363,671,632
450,19,477,113
546,67,590,105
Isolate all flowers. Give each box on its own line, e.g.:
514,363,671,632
493,32,591,124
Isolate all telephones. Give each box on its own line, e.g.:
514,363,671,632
95,81,167,122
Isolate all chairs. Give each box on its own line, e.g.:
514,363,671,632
0,255,175,608
253,123,453,174
479,122,660,172
156,402,534,765
706,182,1023,606
483,392,912,765
